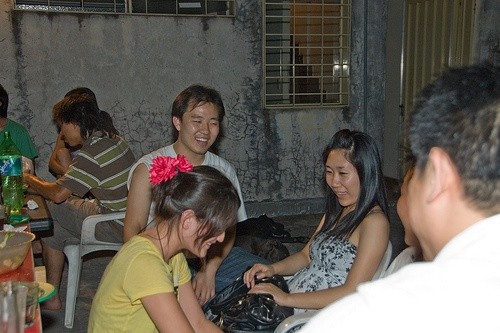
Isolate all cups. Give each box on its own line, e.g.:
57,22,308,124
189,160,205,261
0,280,39,333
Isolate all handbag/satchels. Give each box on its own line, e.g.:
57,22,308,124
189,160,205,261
235,214,291,242
200,267,294,333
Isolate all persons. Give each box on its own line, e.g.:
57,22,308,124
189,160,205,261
296,62,500,333
0,85,500,333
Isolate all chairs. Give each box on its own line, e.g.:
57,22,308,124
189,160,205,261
274,241,393,333
63,212,128,329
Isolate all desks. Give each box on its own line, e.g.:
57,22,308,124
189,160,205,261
0,188,56,333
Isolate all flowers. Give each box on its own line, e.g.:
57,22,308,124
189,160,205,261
148,154,193,185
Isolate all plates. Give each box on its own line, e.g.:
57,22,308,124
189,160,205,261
39,282,57,302
6,214,32,226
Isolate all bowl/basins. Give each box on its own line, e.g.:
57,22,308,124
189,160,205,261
0,230,36,274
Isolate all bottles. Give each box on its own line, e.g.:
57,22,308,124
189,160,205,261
0,130,24,211
